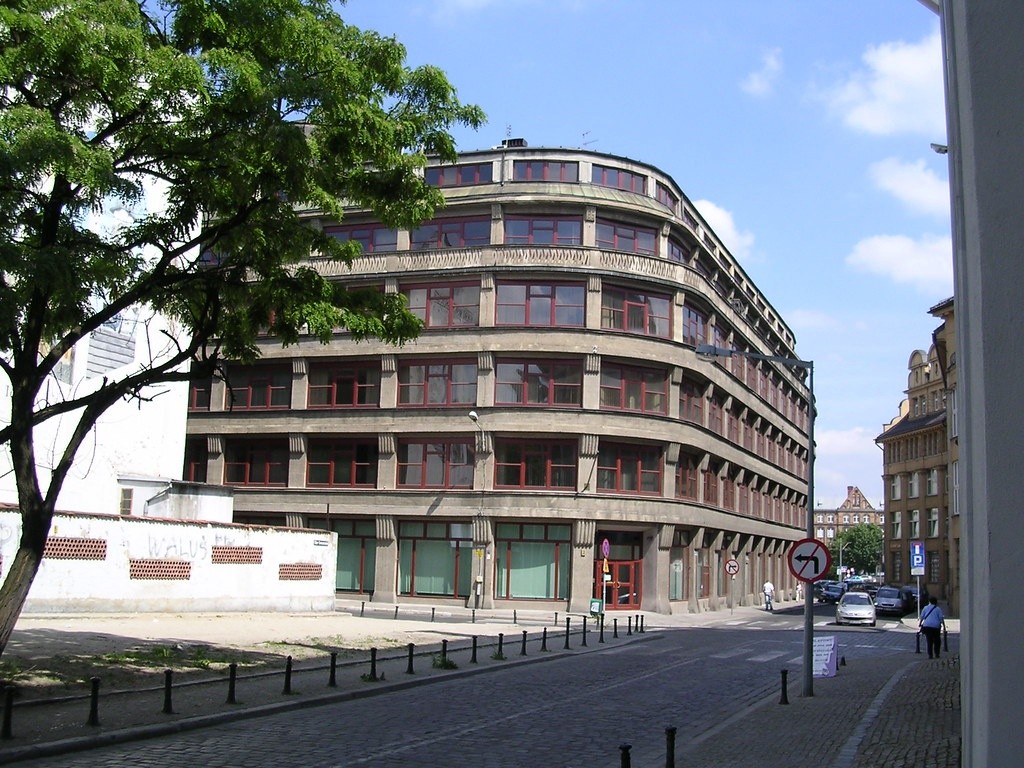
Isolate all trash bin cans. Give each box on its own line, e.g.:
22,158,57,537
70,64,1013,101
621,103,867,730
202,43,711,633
590,598,602,616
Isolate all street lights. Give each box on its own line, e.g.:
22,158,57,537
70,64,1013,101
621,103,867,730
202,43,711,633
694,341,818,698
838,538,853,582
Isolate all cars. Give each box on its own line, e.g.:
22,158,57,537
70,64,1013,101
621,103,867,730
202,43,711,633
834,591,879,627
813,573,926,618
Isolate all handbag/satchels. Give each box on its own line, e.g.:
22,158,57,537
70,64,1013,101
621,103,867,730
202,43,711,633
942,630,948,652
920,625,924,635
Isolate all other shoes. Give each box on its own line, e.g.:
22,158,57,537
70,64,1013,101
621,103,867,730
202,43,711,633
770,608,773,610
766,608,768,610
929,655,933,659
936,656,939,658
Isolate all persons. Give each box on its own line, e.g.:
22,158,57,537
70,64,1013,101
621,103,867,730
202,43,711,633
919,597,946,660
796,580,802,603
762,580,776,610
